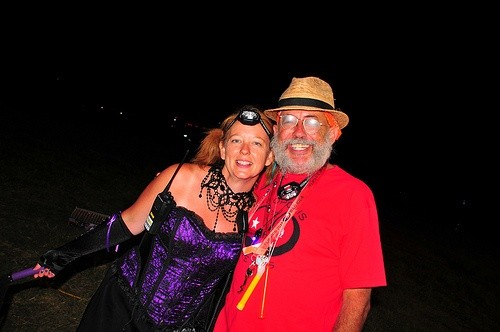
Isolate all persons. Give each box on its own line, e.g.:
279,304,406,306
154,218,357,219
32,107,274,332
204,76,387,332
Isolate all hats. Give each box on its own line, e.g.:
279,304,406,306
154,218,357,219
264,77,350,129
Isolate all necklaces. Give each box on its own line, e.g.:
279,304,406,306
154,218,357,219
238,173,314,290
199,165,252,233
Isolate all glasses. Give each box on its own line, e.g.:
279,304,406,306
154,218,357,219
279,114,328,135
